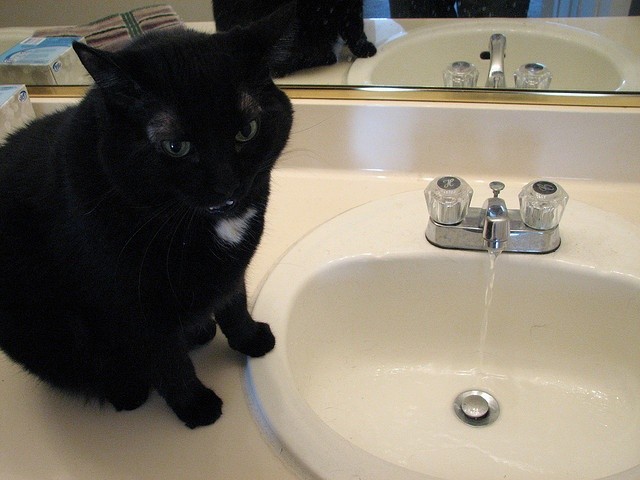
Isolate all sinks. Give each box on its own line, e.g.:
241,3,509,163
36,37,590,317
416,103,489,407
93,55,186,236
367,29,624,92
239,253,639,479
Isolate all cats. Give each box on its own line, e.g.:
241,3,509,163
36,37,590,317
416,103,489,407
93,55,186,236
212,0,377,79
0,16,324,429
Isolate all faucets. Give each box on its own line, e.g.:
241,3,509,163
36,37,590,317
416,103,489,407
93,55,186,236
483,34,508,89
472,176,517,254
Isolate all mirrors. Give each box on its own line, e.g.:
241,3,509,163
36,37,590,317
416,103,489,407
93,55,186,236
0,0,640,109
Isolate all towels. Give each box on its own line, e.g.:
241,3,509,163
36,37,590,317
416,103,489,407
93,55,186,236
34,5,183,53
